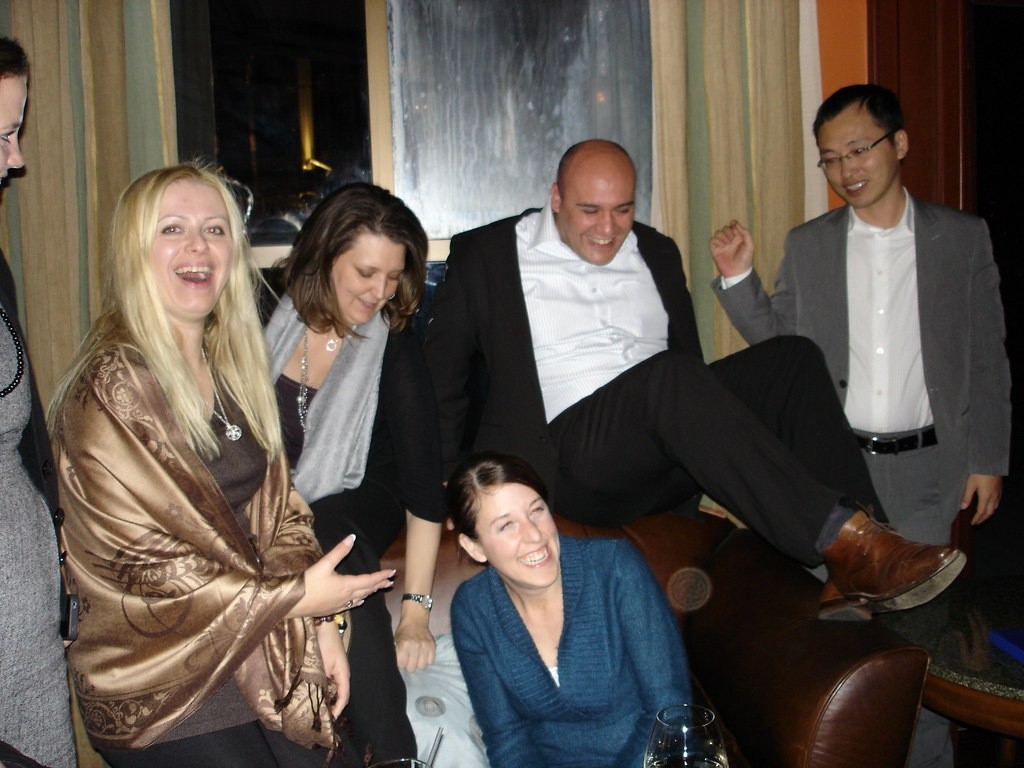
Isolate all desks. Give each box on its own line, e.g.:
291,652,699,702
874,575,1024,768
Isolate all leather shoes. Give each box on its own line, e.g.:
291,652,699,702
821,511,966,611
818,577,872,620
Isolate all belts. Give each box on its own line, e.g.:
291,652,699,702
851,428,937,455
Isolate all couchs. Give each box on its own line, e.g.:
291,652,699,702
380,512,932,768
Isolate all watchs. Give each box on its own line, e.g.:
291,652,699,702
400,592,433,612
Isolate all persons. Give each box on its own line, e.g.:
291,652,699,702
708,84,1012,768
0,36,77,768
446,450,695,768
48,167,398,768
247,182,444,768
229,149,372,329
424,138,966,622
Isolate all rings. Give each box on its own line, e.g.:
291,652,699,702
346,600,353,608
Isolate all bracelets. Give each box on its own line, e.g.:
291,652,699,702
314,614,347,638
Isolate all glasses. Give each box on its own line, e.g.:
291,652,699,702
817,131,896,173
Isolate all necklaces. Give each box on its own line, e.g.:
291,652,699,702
0,308,24,397
296,331,310,429
199,347,241,441
